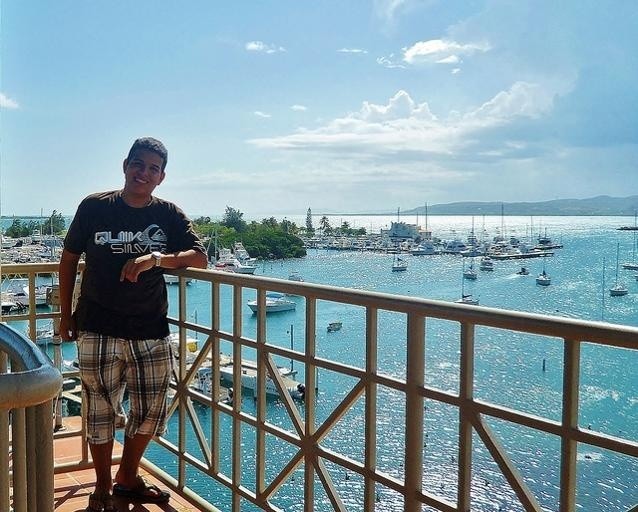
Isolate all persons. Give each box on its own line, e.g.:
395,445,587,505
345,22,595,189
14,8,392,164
58,137,209,512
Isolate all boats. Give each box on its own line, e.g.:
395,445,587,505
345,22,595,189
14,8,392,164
326,320,342,332
247,298,297,314
609,212,638,298
0,207,63,308
294,200,563,276
455,259,479,305
163,227,258,285
268,271,306,297
24,307,319,426
536,248,551,286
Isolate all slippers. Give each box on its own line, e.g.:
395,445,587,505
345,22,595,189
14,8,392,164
112,475,171,504
89,490,121,512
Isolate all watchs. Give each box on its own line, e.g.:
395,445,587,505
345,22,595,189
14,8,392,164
151,250,162,267
